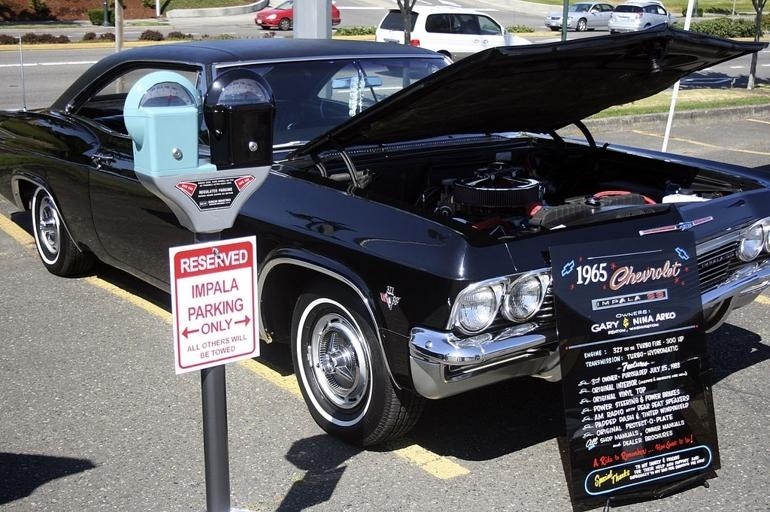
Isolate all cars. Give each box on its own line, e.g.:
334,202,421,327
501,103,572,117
544,2,616,32
255,1,341,30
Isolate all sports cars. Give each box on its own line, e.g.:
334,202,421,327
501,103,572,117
0,17,770,454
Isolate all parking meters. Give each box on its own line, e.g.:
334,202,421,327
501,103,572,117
124,69,277,512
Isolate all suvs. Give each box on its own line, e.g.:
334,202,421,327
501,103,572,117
373,6,535,76
608,2,678,35
